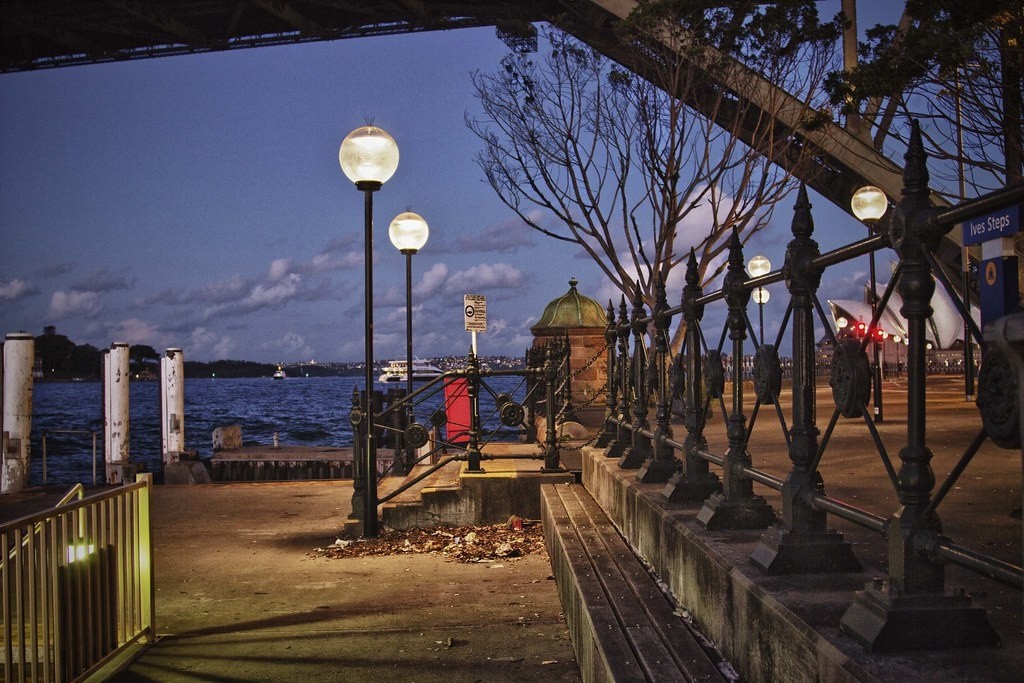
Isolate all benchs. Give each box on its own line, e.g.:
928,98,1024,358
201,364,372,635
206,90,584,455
539,481,725,683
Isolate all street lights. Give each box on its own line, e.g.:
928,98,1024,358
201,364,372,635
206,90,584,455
893,335,902,378
747,254,772,344
339,124,401,537
387,210,430,475
850,183,889,423
883,331,888,379
836,317,848,338
926,343,933,374
938,56,984,401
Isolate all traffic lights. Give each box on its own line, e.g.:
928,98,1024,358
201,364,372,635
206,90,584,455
857,323,865,337
877,329,883,342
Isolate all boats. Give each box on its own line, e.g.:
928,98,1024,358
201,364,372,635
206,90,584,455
273,371,286,380
377,353,444,383
